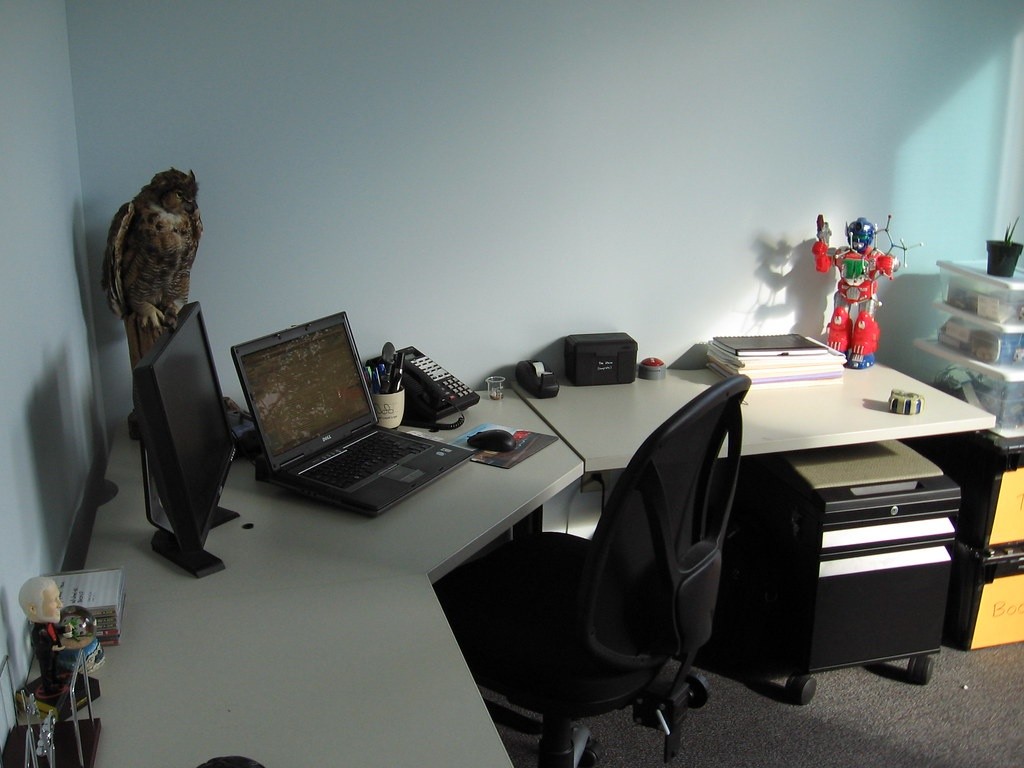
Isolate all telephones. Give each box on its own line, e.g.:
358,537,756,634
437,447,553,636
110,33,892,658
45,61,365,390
364,346,481,429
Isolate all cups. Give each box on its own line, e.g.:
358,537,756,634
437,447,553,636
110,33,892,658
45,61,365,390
486,376,505,400
369,386,405,428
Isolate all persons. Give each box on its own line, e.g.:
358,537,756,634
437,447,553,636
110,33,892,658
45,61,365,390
17,577,74,698
812,213,900,371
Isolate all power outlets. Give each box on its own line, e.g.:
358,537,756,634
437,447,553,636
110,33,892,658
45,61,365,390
580,470,610,493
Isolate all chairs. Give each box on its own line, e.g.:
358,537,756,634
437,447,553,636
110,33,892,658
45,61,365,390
434,373,750,768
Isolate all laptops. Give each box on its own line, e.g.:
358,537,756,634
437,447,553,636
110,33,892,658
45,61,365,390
231,312,476,514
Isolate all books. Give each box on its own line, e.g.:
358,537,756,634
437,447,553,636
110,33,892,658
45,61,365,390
705,330,847,389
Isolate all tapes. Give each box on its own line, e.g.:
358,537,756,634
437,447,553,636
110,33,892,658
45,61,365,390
532,360,546,378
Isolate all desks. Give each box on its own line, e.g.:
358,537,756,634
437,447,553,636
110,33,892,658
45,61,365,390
509,363,996,540
67,388,584,768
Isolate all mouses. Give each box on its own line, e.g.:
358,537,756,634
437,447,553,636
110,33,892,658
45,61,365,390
467,429,516,452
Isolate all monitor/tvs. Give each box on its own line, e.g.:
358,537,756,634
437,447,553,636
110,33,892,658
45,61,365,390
127,301,240,578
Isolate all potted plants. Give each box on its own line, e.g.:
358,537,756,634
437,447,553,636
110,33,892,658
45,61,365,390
985,216,1024,278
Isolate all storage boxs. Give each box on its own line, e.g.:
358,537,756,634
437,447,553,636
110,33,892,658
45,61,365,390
933,297,1024,370
936,259,1024,325
913,336,1024,437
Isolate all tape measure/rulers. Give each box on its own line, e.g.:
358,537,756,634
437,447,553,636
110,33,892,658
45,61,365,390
887,388,926,415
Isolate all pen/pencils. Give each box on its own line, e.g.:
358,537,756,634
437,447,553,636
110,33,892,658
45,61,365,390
361,351,407,394
